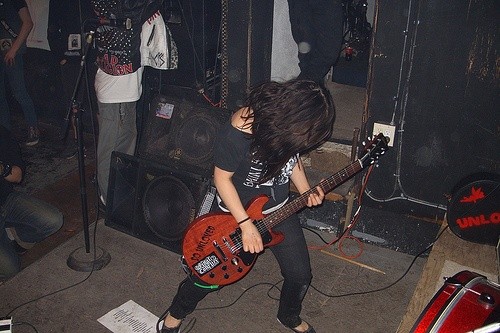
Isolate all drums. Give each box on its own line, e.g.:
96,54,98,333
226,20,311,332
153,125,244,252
408,266,499,333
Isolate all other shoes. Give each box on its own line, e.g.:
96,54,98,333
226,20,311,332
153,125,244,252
26,125,41,146
96,197,106,212
161,312,180,332
2,227,24,254
277,315,316,333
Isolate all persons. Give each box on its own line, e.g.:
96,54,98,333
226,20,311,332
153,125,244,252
92,0,179,213
161,78,336,333
0,125,63,284
0,0,42,145
252,0,344,82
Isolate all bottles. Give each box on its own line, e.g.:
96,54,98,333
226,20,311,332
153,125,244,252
72,100,78,119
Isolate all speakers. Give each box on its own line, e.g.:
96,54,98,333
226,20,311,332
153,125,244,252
102,151,226,256
133,90,238,183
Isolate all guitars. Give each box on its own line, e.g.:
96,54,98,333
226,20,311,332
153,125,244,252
182,132,390,287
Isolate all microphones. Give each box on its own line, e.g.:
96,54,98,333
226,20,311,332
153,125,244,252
84,17,122,28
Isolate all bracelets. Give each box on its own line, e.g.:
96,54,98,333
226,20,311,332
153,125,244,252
0,160,12,177
238,217,250,225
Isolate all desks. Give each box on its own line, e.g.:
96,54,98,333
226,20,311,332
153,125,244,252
393,207,500,333
